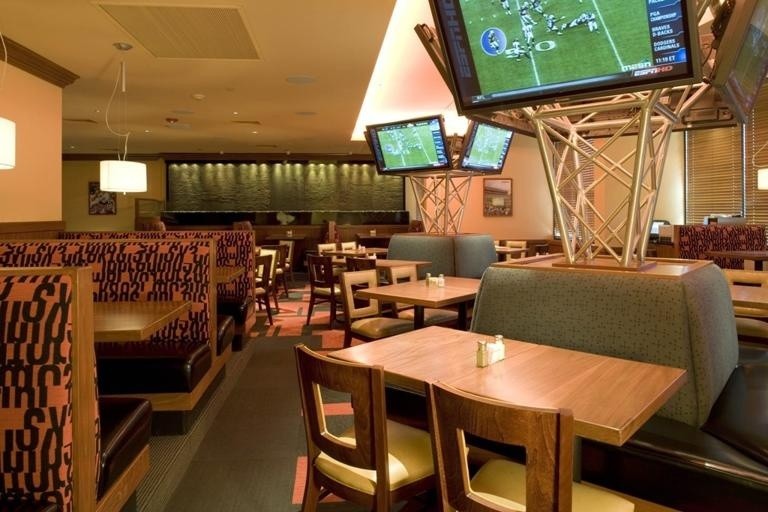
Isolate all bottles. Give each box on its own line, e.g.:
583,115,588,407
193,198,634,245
425,272,432,287
474,339,489,368
492,333,507,362
438,274,445,288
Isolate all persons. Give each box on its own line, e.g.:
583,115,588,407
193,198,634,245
486,0,601,61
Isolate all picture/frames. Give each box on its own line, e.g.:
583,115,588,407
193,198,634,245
482,177,514,217
87,181,119,215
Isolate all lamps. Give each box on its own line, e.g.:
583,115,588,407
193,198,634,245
97,41,149,199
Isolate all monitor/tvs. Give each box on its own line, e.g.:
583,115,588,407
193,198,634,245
460,121,513,175
706,1,767,121
650,219,667,241
367,115,450,176
430,0,702,114
415,24,573,142
